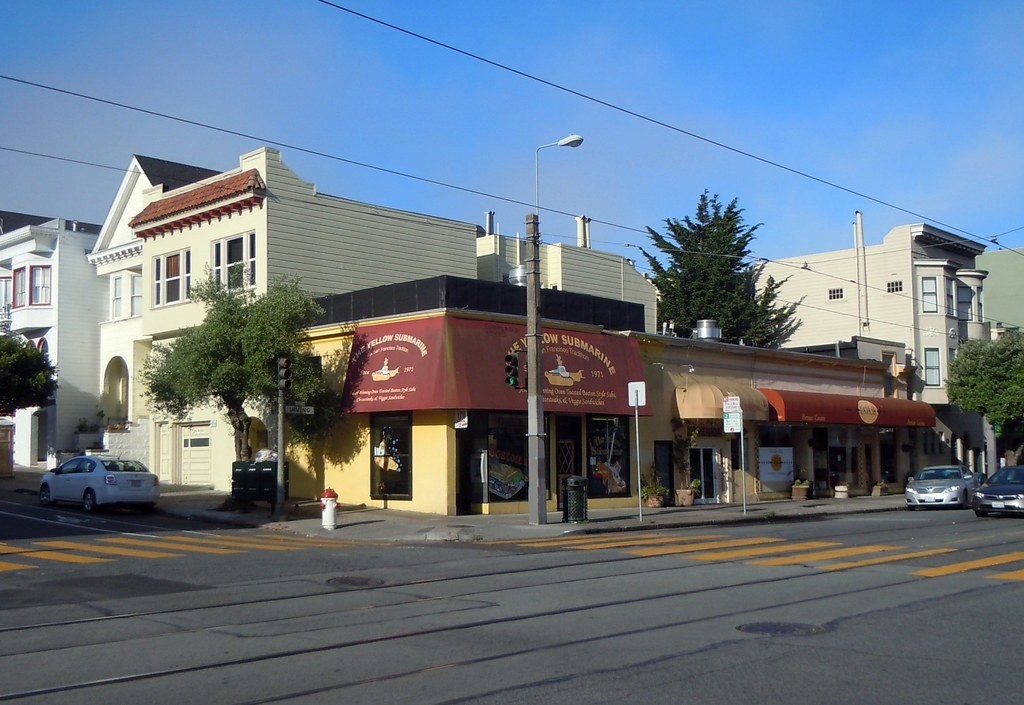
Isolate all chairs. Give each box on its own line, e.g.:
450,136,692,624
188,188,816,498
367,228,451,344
106,463,136,471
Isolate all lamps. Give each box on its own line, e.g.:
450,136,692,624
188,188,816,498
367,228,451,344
681,364,695,373
652,362,664,371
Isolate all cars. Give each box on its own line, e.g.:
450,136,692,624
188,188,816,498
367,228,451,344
973,465,1024,518
904,464,977,509
38,455,160,512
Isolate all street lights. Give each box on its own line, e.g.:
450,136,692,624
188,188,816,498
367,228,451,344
508,135,585,527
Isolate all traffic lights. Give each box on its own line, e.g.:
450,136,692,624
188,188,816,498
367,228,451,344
505,353,519,386
277,354,292,390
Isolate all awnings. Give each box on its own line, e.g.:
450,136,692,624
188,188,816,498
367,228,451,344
339,317,653,416
758,388,936,426
676,381,769,423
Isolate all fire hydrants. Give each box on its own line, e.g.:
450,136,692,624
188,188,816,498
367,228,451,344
318,488,341,529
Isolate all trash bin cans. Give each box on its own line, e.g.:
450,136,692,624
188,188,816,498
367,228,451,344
562,475,589,522
232,461,289,501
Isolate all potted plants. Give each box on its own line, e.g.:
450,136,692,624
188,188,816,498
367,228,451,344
871,481,889,497
674,478,703,507
636,466,670,508
791,480,810,501
834,482,849,498
